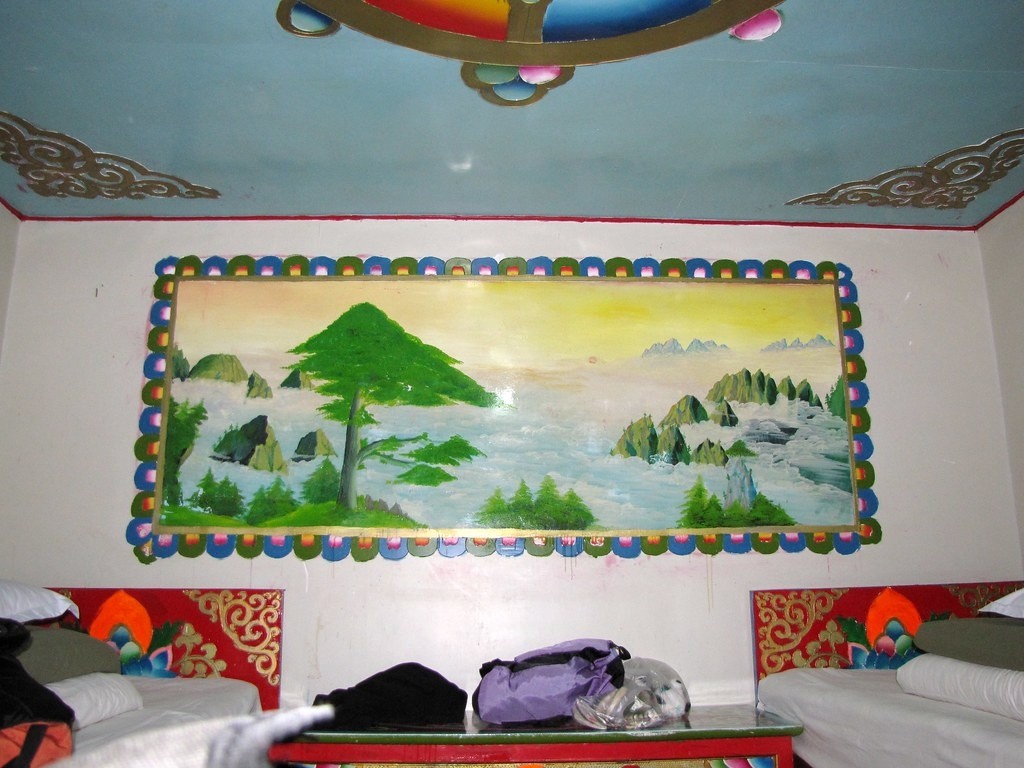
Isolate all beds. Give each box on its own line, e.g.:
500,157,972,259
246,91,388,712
0,586,286,768
747,578,1024,768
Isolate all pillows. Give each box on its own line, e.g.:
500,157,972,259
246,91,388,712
0,584,82,627
976,588,1024,621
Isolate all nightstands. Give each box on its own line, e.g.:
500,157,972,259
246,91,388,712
263,701,807,768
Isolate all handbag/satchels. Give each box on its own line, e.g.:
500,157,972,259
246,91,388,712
570,657,691,730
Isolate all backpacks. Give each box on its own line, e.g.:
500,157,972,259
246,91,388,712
472,638,630,727
311,662,468,733
0,654,75,768
